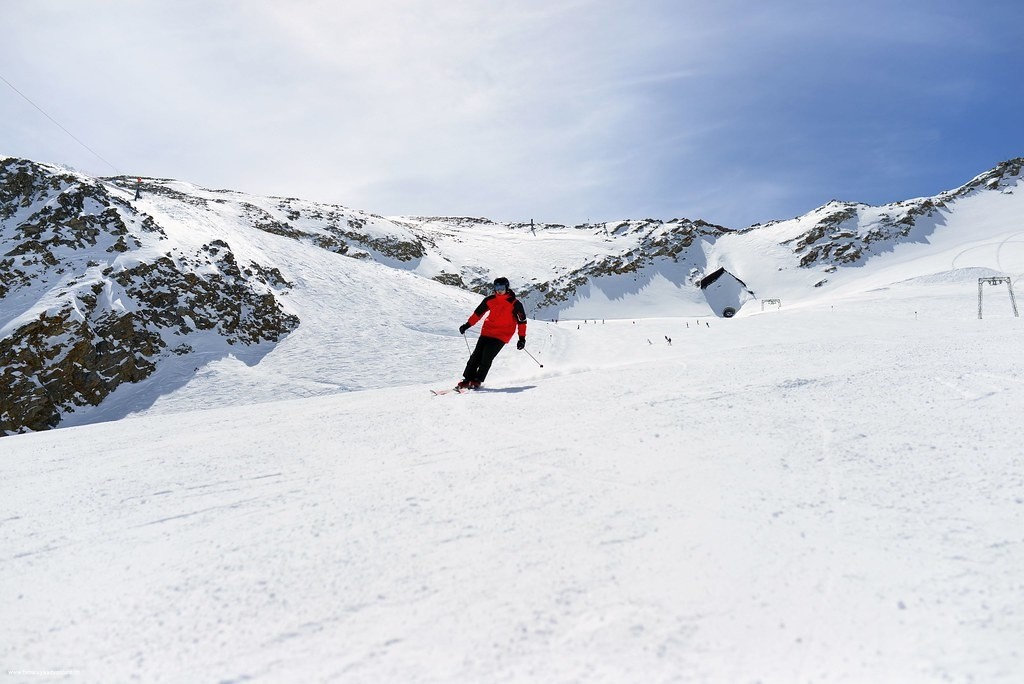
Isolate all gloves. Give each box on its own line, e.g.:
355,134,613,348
517,336,526,350
459,322,472,334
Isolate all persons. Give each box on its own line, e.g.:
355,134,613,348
454,276,527,391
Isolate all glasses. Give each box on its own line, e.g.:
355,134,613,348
495,286,505,291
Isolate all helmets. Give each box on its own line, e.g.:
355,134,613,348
494,277,510,292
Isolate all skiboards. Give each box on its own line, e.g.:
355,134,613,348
429,385,485,395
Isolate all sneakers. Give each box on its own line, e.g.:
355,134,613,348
454,379,481,393
